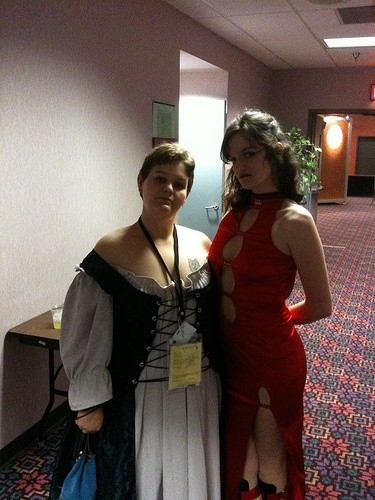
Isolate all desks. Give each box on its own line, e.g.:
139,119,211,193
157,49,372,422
8,310,68,443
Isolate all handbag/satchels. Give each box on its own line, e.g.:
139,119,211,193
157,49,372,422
58,432,97,500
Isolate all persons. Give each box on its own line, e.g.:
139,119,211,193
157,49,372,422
51,143,230,500
208,109,332,500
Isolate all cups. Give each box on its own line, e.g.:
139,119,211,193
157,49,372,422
50,304,63,330
